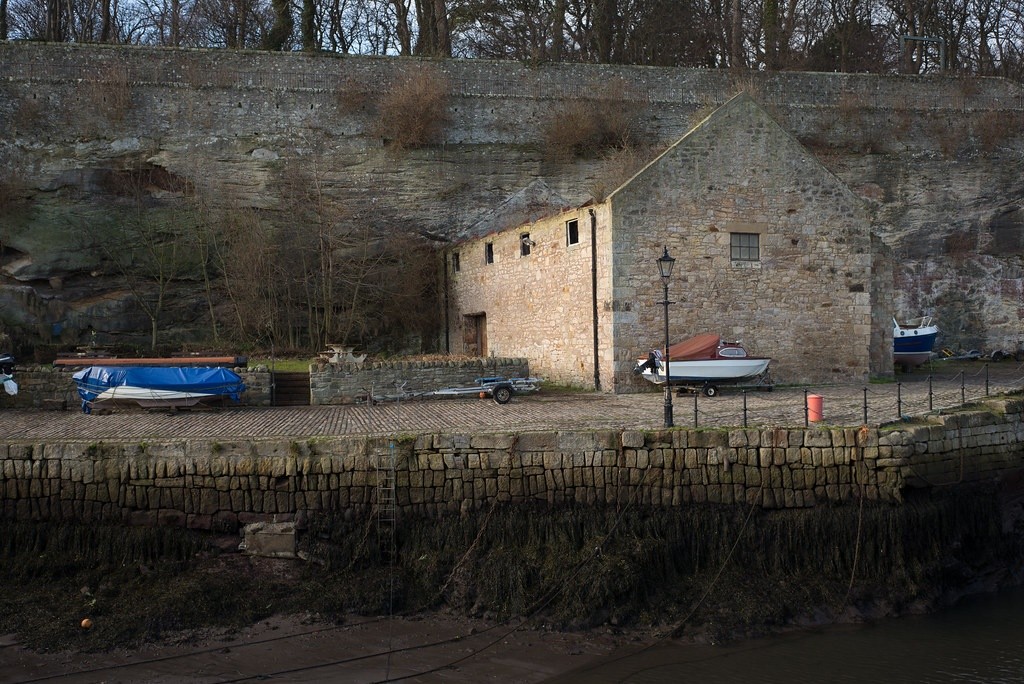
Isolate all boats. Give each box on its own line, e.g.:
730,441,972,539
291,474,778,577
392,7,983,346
71,365,247,414
634,333,772,395
893,314,941,372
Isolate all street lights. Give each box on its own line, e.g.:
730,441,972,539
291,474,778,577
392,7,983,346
654,244,676,429
